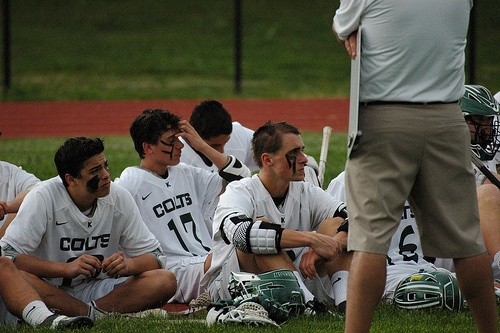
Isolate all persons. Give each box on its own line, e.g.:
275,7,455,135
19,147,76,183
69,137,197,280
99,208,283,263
0,0,500,332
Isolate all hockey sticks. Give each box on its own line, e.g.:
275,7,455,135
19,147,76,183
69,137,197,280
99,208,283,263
471,150,500,190
317,126,332,187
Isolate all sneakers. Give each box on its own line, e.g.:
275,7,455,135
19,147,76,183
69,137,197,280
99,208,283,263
34,314,93,331
303,297,342,322
108,309,168,324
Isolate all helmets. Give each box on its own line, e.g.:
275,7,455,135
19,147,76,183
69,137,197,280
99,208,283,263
458,85,500,161
227,269,305,326
393,272,464,314
